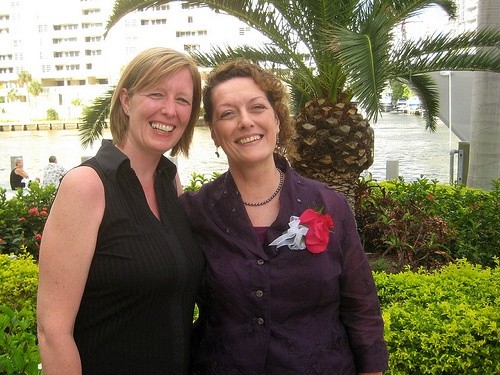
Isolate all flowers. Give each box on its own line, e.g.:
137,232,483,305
299,203,335,253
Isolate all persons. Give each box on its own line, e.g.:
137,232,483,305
43,156,68,190
177,60,389,375
10,158,29,190
37,47,202,375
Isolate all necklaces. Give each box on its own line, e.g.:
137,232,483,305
243,168,283,206
